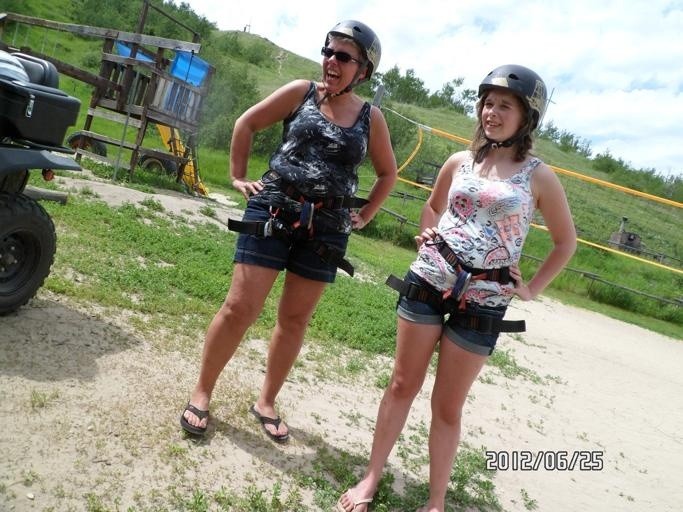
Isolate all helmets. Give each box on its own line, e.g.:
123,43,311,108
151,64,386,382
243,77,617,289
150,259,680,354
323,19,381,86
477,63,548,133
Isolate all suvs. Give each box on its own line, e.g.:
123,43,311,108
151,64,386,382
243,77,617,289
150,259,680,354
1,48,83,314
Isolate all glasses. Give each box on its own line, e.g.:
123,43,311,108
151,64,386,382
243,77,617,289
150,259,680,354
320,46,365,65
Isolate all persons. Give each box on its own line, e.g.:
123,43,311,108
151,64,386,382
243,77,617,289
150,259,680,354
181,21,397,442
337,65,578,512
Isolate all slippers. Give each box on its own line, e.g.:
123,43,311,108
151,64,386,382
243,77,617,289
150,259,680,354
180,399,210,436
250,404,289,444
336,483,381,512
